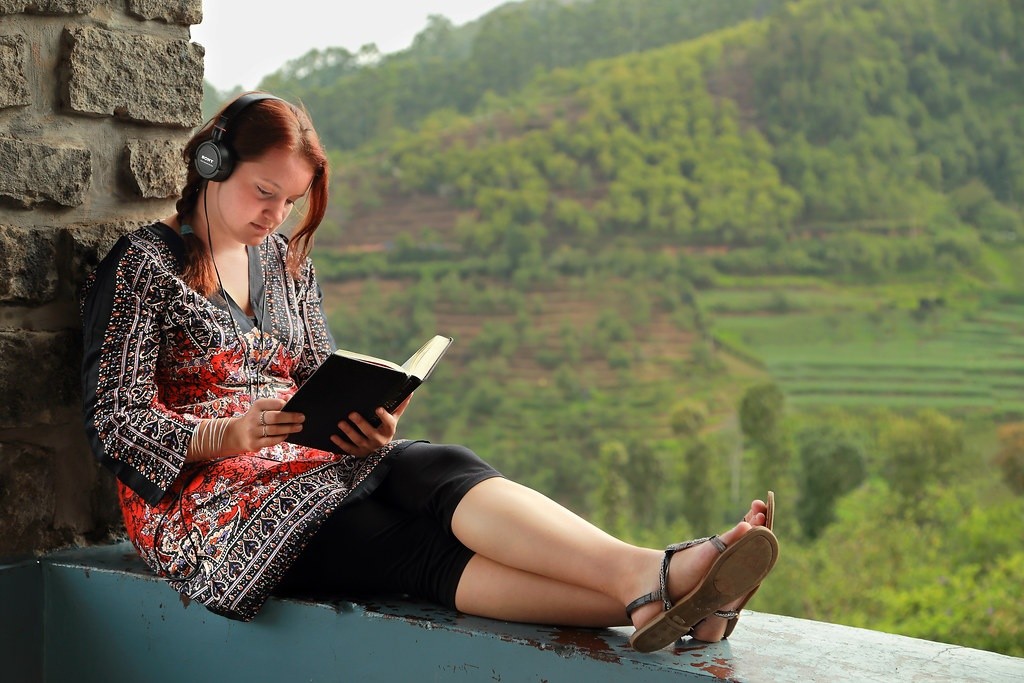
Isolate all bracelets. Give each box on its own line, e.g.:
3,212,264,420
191,418,231,458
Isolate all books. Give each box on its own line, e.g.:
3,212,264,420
278,334,452,454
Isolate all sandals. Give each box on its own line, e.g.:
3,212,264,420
625,524,779,655
685,488,774,638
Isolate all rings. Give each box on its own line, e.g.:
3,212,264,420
260,410,266,425
262,426,267,437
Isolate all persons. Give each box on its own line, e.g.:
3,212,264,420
73,91,779,654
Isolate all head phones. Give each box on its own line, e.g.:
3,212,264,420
195,94,281,182
